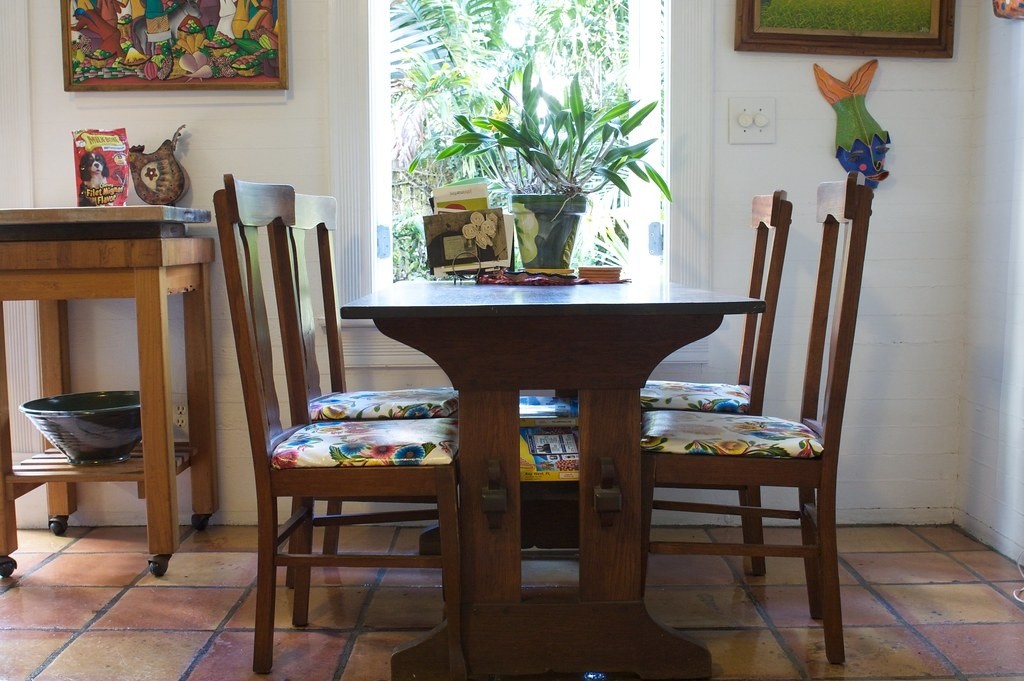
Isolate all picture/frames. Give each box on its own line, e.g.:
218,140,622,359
60,0,288,93
734,0,956,59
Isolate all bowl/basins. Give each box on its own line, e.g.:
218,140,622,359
19,389,142,464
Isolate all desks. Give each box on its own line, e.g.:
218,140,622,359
0,205,214,580
341,280,768,681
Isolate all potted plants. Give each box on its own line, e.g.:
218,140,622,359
438,60,673,275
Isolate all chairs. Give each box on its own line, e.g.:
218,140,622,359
641,189,793,578
284,194,460,589
212,174,467,677
640,180,877,666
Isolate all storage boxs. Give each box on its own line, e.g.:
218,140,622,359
520,395,578,428
520,426,582,550
